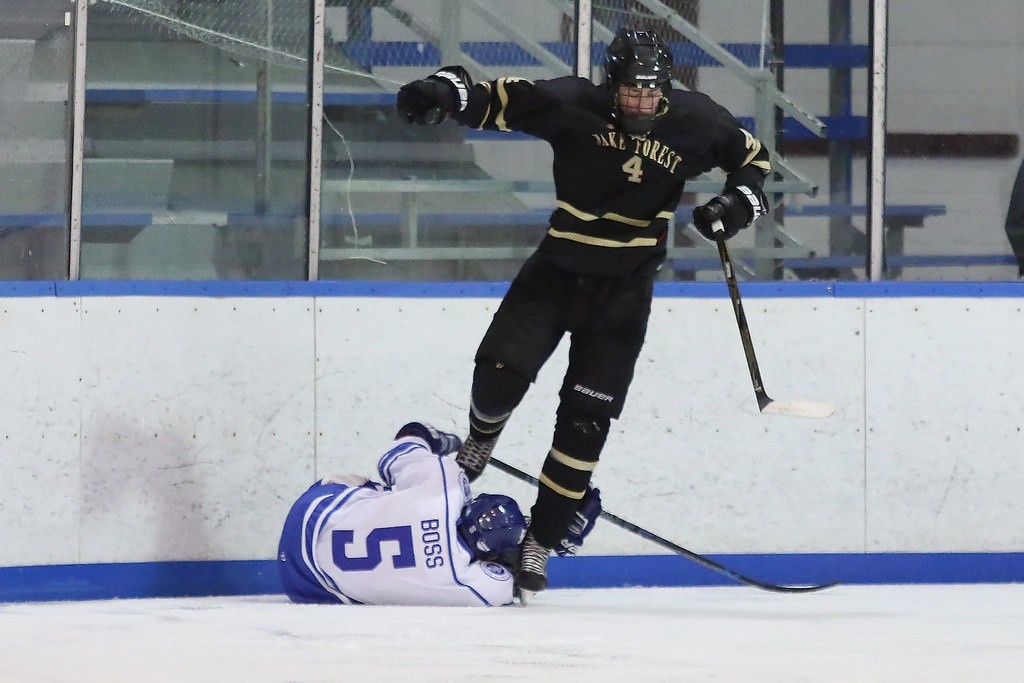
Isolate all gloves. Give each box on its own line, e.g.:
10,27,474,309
397,65,474,126
692,183,769,241
393,420,461,456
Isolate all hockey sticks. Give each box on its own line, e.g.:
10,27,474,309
458,443,844,595
711,218,839,422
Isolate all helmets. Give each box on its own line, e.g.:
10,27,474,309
603,27,673,131
455,493,528,573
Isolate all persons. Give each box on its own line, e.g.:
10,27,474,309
398,28,772,605
276,418,602,606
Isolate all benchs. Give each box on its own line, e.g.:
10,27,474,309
0,0,1019,280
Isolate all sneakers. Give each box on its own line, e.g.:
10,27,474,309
455,433,500,483
517,527,552,592
